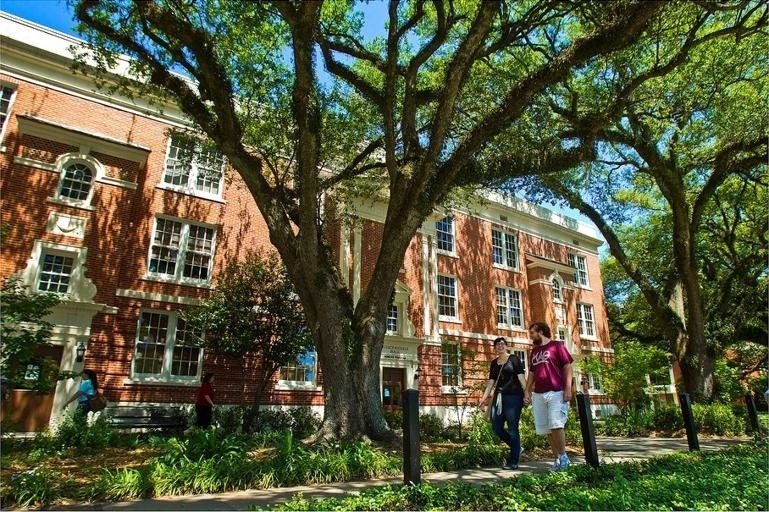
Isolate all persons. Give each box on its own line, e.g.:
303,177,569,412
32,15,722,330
191,372,217,430
521,322,577,473
478,336,532,470
59,369,100,423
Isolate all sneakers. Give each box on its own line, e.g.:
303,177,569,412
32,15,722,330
502,465,519,470
548,460,573,473
519,446,524,454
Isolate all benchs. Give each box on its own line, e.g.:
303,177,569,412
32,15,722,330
103,405,187,442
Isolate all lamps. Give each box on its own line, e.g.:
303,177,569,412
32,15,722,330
76,340,86,362
414,366,422,379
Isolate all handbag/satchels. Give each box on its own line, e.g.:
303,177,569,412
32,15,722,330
196,403,211,421
86,394,105,411
484,397,493,423
